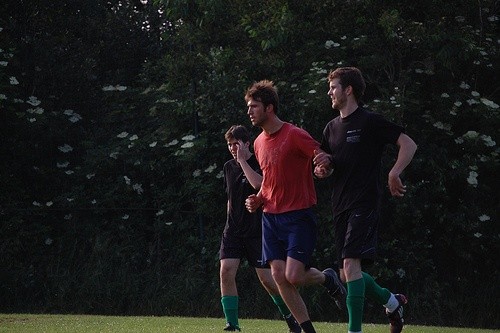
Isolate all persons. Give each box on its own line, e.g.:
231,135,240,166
219,124,302,333
245,81,347,333
312,67,417,333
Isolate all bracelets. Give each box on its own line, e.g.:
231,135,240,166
327,154,333,166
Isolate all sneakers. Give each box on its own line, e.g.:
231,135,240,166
290,324,301,333
224,325,240,332
322,268,348,310
387,294,408,333
